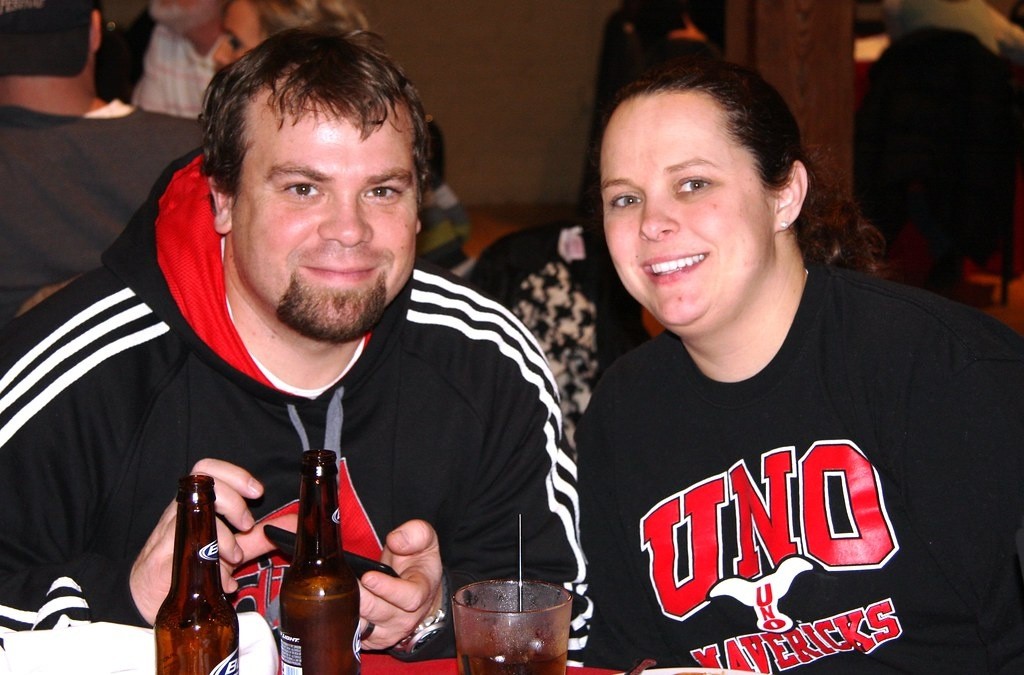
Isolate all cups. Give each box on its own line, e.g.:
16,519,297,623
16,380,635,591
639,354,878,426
452,579,573,675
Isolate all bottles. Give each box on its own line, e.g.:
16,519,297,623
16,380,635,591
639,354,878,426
153,475,239,675
279,446,361,675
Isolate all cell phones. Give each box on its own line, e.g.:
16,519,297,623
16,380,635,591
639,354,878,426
263,524,400,580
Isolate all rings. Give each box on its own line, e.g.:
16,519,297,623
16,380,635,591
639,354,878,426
360,622,375,641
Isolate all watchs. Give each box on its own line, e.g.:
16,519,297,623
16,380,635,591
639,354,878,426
394,609,448,656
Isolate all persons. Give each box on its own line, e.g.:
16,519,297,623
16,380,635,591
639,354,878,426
572,57,1024,675
0,0,595,675
852,0,1024,332
472,0,724,431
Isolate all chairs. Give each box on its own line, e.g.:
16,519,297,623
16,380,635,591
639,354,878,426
854,25,1024,306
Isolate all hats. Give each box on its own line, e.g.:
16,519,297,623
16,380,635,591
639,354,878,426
0,0,103,76
